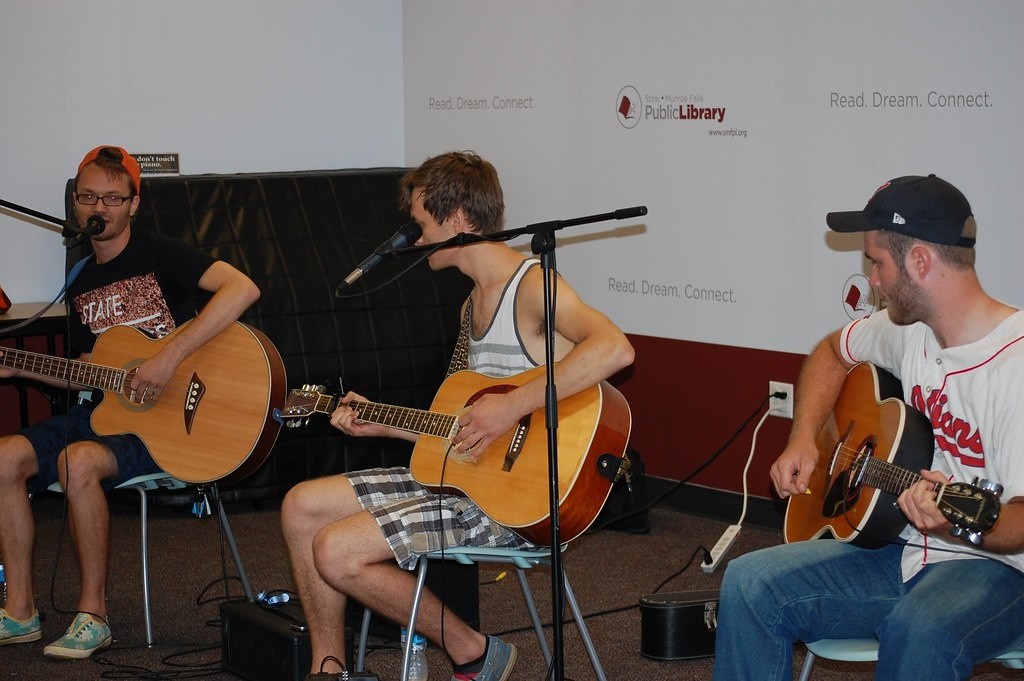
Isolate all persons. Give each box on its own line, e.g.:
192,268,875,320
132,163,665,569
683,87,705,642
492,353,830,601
0,144,260,657
713,173,1024,681
279,150,635,681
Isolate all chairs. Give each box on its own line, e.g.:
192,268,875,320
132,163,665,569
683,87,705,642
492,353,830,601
29,308,256,650
798,638,1024,681
356,543,607,681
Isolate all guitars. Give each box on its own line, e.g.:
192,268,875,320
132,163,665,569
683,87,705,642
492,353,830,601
0,316,287,486
279,361,632,546
784,362,1004,550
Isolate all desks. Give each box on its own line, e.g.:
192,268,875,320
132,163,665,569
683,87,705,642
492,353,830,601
0,301,71,427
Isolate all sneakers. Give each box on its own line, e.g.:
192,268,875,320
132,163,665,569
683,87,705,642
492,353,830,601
44,611,113,658
0,607,43,645
453,634,518,681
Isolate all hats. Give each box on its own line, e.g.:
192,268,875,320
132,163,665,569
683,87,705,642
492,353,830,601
826,173,977,249
76,144,141,194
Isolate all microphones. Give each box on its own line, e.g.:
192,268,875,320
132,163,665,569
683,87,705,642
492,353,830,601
337,220,424,291
74,215,106,243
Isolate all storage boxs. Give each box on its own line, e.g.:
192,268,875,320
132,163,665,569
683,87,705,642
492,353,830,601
637,588,721,661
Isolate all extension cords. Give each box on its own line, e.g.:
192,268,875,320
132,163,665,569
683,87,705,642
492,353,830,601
701,524,743,573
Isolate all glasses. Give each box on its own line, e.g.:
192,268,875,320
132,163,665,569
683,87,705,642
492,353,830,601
75,189,133,207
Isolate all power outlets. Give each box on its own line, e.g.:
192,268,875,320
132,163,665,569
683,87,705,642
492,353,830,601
768,380,794,420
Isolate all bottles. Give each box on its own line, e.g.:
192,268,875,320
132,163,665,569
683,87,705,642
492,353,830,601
401,627,432,681
0,560,7,609
